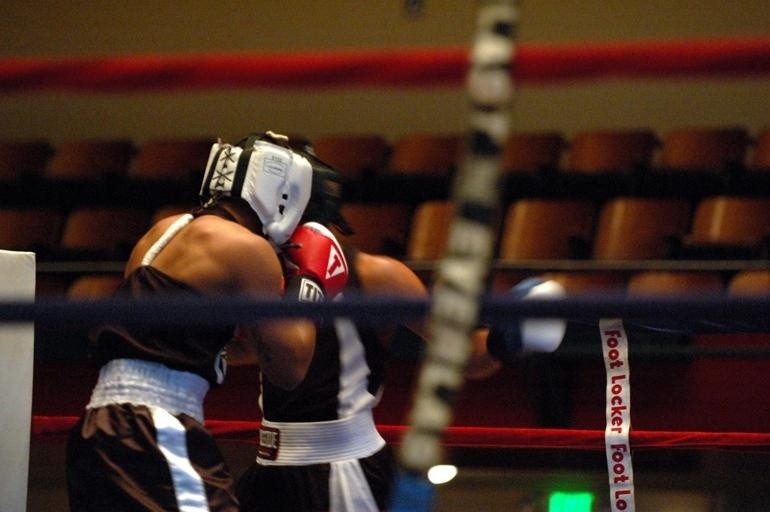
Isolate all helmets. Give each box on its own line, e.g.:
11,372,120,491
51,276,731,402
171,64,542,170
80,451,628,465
197,132,340,247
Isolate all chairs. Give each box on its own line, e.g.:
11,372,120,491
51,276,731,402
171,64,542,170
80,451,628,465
2,119,768,467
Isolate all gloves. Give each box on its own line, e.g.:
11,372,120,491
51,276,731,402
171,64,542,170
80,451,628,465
279,221,348,303
487,277,569,357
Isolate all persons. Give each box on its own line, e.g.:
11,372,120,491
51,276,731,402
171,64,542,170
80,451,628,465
65,132,349,512
235,139,568,512
